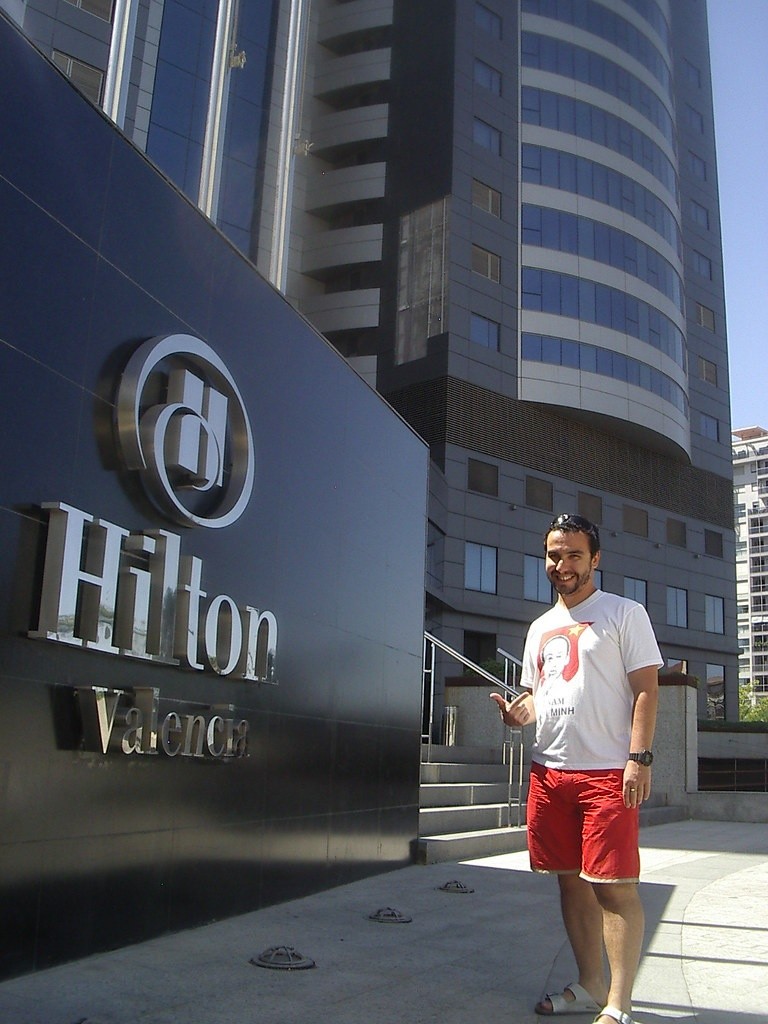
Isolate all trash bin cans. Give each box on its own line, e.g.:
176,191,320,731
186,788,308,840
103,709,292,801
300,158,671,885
441,706,458,746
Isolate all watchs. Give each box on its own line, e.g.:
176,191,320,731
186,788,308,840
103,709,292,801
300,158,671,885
629,750,654,766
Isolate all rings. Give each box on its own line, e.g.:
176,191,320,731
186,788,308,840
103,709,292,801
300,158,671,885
631,789,636,792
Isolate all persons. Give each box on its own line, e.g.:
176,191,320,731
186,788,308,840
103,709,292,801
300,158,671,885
490,514,664,1024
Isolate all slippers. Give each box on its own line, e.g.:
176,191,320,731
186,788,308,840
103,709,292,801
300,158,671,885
592,1006,632,1024
535,982,604,1015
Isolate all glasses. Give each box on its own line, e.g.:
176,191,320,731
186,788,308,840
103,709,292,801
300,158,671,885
553,513,597,538
